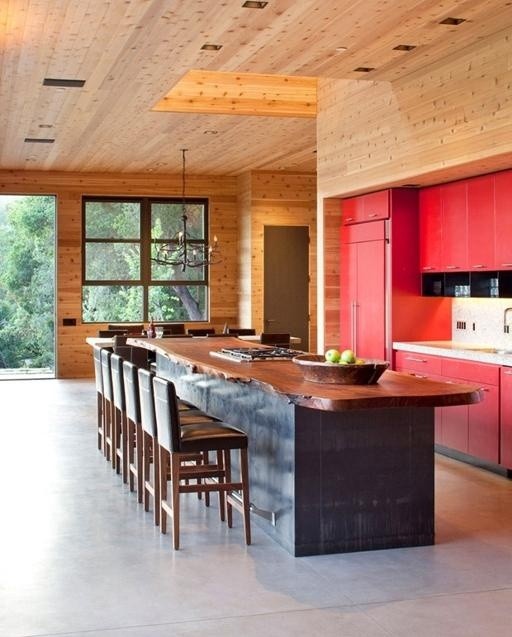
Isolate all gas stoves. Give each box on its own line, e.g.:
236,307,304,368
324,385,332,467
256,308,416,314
210,345,301,367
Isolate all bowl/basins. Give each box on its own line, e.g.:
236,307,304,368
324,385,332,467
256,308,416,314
292,353,391,385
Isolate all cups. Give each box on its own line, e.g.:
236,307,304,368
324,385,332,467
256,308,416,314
154,326,163,339
146,329,154,339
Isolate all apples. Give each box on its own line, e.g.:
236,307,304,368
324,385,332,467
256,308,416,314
325,349,365,364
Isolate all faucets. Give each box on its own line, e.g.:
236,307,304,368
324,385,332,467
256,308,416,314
503,308,512,334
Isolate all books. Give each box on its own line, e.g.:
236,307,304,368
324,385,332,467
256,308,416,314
207,345,306,363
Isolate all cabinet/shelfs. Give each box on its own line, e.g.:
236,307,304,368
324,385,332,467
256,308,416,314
468,167,512,272
419,174,469,272
393,350,442,457
340,187,419,226
443,355,500,477
334,219,453,371
501,365,512,477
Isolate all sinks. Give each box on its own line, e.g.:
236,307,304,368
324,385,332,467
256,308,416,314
463,348,512,355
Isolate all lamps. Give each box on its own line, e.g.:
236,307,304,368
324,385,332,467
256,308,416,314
147,147,224,273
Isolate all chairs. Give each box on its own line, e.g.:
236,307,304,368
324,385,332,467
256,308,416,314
136,367,228,526
151,377,253,551
85,321,305,348
91,345,156,475
120,358,204,504
